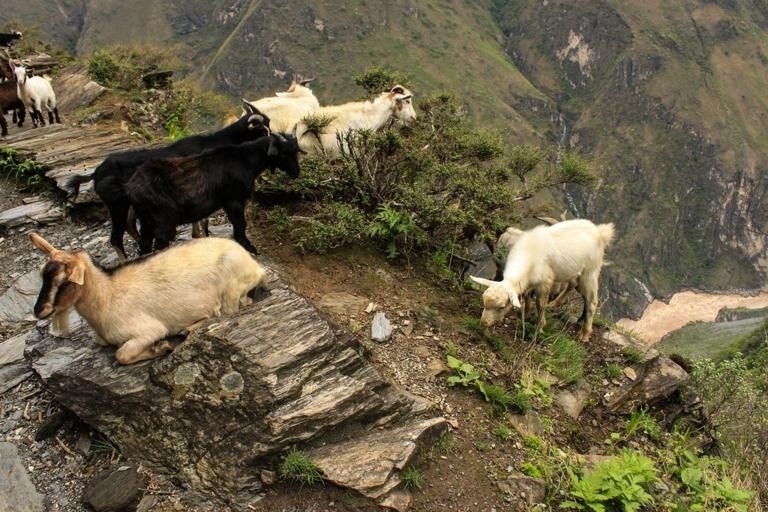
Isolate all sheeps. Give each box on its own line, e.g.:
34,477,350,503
213,76,323,136
26,230,271,365
0,30,61,136
63,97,308,265
294,81,418,160
468,209,617,343
